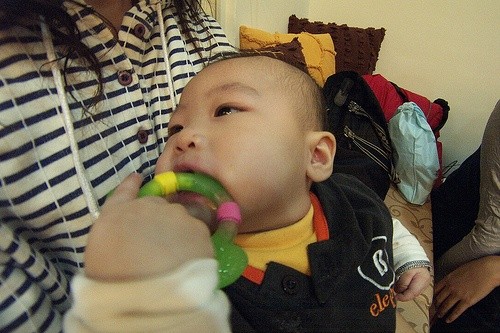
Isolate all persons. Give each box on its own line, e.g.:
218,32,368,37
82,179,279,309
63,51,432,333
0,0,239,332
431,97,500,329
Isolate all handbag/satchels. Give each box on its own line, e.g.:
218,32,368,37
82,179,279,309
389,100,438,206
321,68,396,205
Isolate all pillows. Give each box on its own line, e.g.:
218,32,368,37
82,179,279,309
239,25,336,90
288,14,387,78
239,37,310,74
